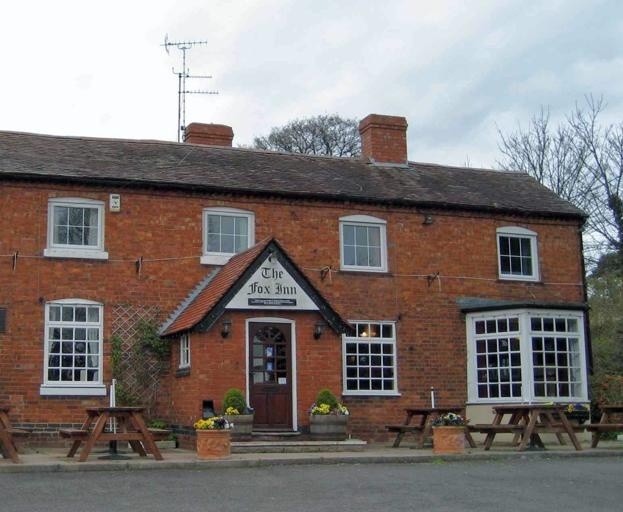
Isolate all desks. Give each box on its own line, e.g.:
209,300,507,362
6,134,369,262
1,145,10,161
392,406,477,449
591,404,623,448
0,407,20,463
482,404,584,452
67,407,165,462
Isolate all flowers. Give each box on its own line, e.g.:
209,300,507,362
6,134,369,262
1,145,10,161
194,416,229,430
225,406,240,415
305,402,350,416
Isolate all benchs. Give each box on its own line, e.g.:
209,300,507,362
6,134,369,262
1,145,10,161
147,428,172,437
60,429,90,441
9,428,29,438
384,423,623,451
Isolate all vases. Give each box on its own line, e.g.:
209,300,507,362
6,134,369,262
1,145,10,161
310,414,349,441
196,430,231,460
222,415,253,441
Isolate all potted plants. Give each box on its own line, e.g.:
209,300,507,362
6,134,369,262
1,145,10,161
429,412,472,455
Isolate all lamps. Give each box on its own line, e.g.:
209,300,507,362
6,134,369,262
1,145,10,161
221,319,232,338
313,320,327,340
359,324,376,337
